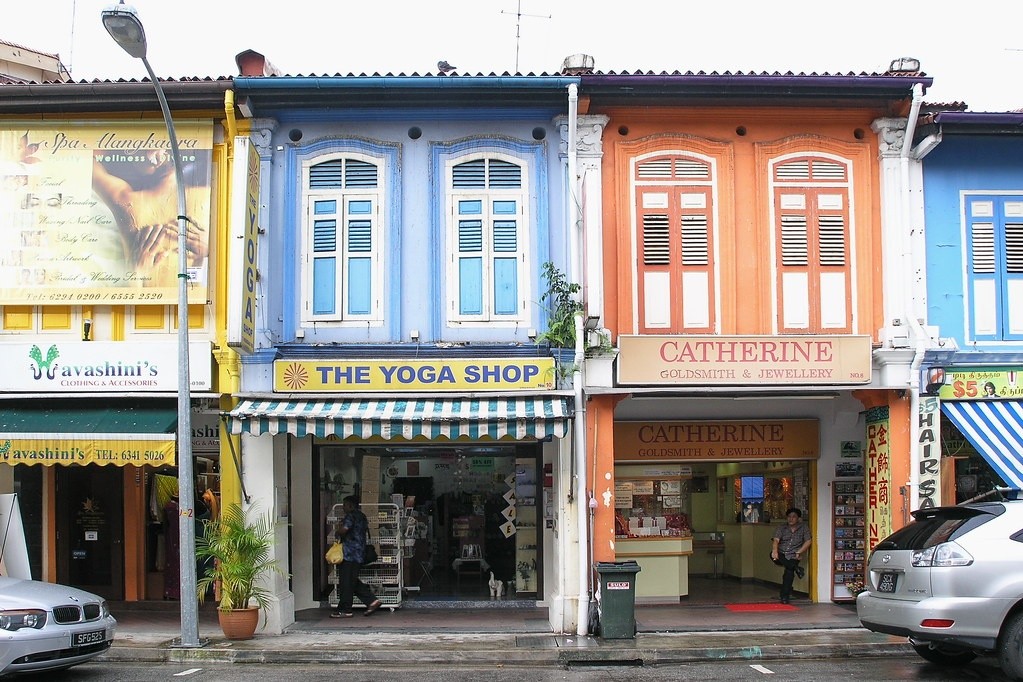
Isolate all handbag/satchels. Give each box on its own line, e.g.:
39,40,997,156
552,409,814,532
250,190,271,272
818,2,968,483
362,526,378,566
325,536,343,564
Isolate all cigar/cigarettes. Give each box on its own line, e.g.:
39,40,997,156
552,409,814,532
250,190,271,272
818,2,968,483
774,558,776,560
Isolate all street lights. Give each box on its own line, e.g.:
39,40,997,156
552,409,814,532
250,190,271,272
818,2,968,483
101,1,211,647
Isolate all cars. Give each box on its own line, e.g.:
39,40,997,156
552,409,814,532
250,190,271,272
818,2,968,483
0,575,118,677
856,500,1023,682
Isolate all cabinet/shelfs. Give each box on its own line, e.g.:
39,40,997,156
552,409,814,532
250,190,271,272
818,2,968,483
327,508,402,608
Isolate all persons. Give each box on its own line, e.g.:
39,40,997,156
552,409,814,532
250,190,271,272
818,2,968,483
770,508,812,604
983,382,1000,398
93,149,208,287
330,495,382,618
744,504,760,523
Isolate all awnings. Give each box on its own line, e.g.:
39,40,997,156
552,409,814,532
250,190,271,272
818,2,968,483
741,477,764,504
941,401,1023,505
0,407,177,467
220,400,576,503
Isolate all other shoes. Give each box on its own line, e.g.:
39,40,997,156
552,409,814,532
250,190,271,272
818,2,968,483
796,566,804,579
781,594,788,604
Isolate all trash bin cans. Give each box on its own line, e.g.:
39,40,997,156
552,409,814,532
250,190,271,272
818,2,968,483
593,560,641,639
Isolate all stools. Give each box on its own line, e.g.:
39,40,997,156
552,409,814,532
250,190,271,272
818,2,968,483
707,550,724,580
416,559,439,588
452,558,490,577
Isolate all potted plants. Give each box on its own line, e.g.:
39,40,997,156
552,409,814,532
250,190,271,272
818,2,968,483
194,498,293,641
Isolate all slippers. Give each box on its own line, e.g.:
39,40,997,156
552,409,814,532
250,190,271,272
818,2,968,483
330,611,354,618
364,601,382,616
